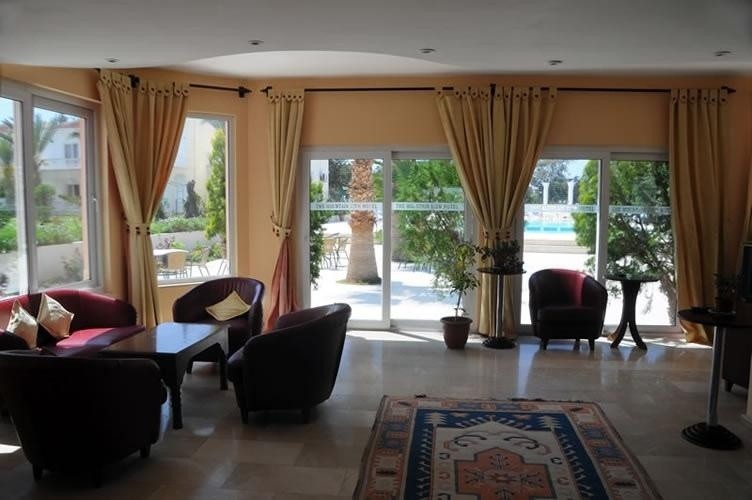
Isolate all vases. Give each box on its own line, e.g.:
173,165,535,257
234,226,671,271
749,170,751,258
491,256,506,270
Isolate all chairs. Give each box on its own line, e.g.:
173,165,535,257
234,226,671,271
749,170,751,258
192,245,215,281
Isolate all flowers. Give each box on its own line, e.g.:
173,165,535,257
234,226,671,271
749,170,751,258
477,227,523,271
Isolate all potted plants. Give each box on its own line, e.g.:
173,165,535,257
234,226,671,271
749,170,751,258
440,240,479,349
713,272,744,311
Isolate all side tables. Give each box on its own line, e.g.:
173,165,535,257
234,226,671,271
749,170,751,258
476,268,526,349
602,274,661,351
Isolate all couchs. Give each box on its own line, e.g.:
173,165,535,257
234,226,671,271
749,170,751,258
0,291,146,360
173,276,266,375
529,268,608,351
1,349,168,489
225,302,350,425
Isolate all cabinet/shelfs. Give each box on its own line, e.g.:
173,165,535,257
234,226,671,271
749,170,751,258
722,294,752,393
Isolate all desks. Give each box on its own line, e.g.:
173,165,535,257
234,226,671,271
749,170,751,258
676,308,744,451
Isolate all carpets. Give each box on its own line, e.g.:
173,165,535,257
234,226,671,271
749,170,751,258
352,395,665,500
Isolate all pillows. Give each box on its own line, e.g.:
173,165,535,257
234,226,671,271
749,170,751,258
38,292,74,340
205,290,252,321
5,299,39,349
